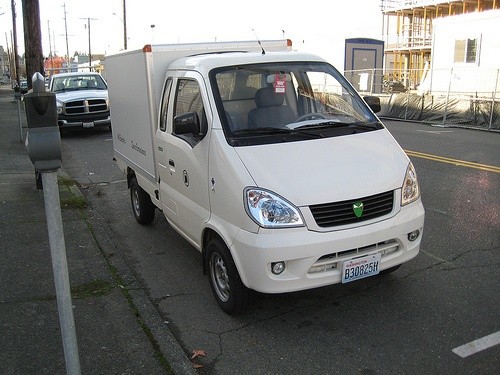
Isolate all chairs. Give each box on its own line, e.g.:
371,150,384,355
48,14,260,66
247,86,297,128
57,83,64,89
201,111,233,133
87,80,97,87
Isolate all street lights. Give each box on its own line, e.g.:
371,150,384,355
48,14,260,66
112,12,127,50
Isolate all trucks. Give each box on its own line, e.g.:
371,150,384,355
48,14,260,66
102,38,426,319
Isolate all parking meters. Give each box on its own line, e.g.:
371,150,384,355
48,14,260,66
22,72,83,375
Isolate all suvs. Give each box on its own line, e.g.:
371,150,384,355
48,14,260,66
45,72,113,133
